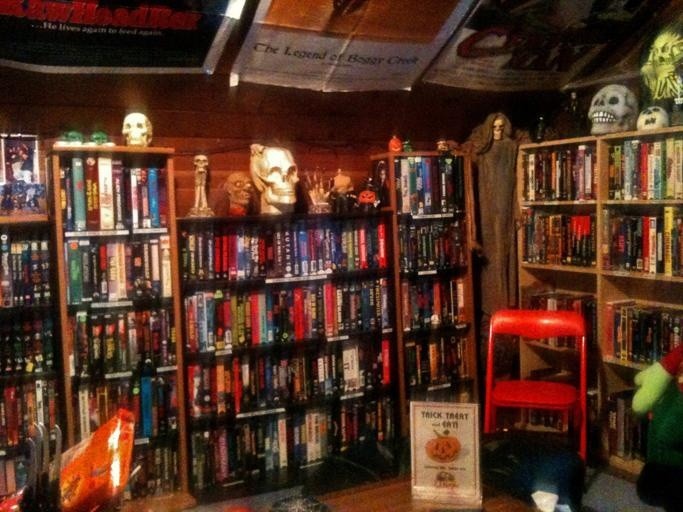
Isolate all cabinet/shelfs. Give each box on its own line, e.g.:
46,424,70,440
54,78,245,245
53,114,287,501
516,127,683,479
371,151,480,430
0,215,68,500
51,145,188,495
177,210,401,503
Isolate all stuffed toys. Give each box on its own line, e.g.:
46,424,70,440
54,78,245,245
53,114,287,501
629,340,683,512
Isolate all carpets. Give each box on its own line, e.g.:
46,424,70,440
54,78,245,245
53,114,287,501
581,472,665,512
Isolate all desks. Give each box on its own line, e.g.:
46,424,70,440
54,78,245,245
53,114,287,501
314,475,543,512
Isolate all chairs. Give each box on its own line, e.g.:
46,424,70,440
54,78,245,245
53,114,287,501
484,309,587,462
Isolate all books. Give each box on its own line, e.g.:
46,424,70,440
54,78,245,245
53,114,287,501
609,137,682,200
602,205,682,277
1,136,180,504
180,218,395,487
604,297,682,365
523,367,597,431
521,143,598,202
604,389,653,464
527,289,595,352
374,155,471,415
520,206,597,268
408,398,483,512
